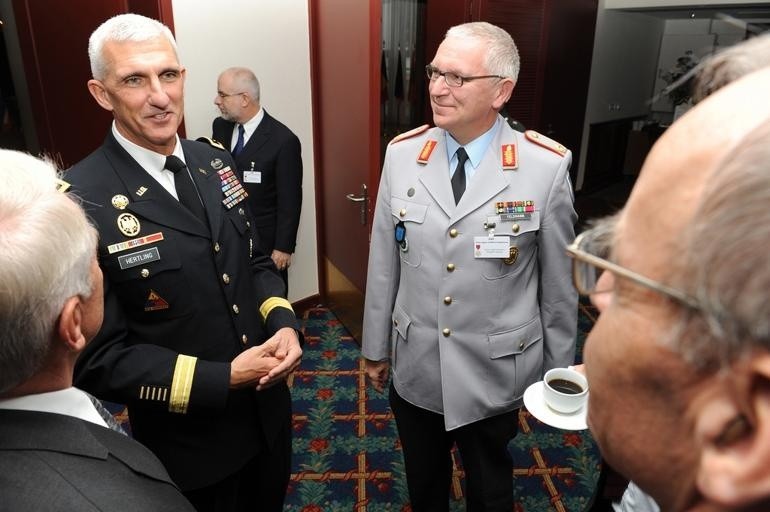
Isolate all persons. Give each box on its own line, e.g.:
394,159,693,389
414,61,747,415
212,67,304,298
54,13,308,512
360,22,579,512
0,146,196,512
566,30,770,512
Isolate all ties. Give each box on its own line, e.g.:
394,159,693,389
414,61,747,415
231,125,245,156
164,156,210,229
451,148,469,206
78,387,131,436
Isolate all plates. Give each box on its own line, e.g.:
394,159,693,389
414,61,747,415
523,381,588,431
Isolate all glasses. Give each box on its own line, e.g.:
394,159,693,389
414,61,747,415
220,92,243,98
426,64,505,87
565,226,704,311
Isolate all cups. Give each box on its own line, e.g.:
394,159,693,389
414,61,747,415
543,364,589,414
